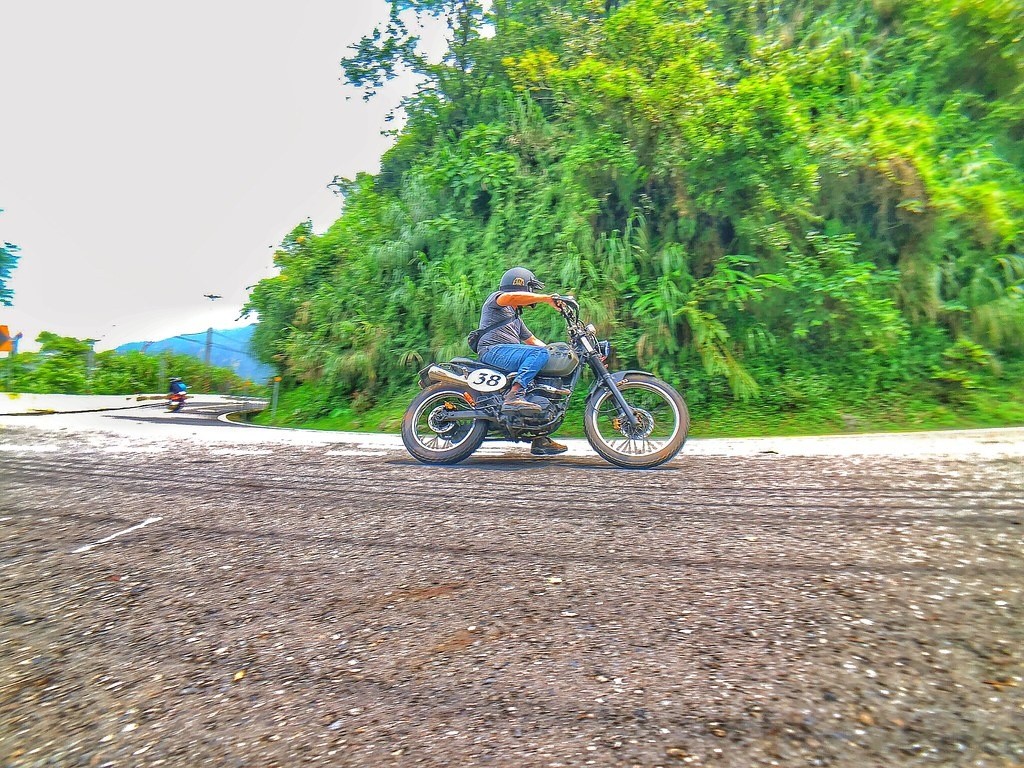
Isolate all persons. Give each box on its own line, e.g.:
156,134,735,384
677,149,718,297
167,378,187,407
476,267,569,456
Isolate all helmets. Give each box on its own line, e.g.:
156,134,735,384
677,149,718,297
499,266,545,308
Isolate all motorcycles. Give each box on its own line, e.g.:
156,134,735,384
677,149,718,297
401,293,690,470
167,391,188,413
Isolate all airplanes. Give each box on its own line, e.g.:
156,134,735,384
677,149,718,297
204,294,222,301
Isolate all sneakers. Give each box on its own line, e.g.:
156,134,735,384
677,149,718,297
501,390,542,416
531,437,568,455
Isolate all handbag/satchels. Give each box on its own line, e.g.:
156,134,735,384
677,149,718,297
468,329,483,353
